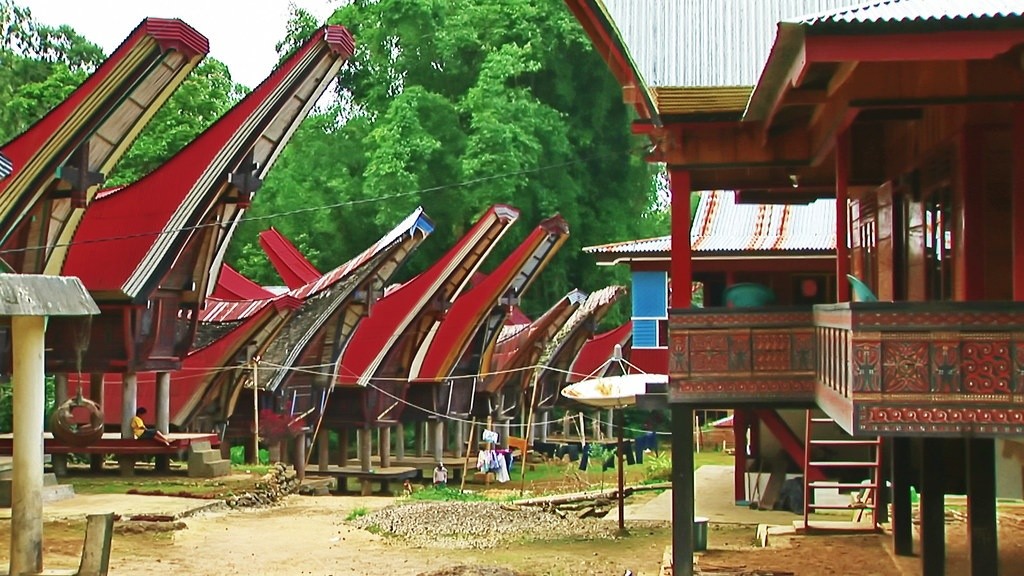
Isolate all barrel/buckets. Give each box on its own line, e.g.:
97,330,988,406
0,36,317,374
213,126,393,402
694,517,708,551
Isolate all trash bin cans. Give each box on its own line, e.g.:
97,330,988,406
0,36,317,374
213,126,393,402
694,517,709,550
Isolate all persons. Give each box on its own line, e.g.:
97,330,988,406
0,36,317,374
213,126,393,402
130,407,171,447
433,461,447,486
